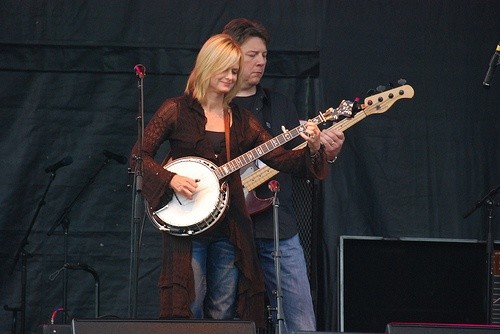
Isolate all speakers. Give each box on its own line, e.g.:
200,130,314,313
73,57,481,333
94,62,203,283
70,318,256,334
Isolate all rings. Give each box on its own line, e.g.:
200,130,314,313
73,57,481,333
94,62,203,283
311,134,316,138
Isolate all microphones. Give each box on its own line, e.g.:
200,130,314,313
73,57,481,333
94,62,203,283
134,63,146,79
102,148,129,165
268,180,280,192
482,42,500,87
45,154,74,173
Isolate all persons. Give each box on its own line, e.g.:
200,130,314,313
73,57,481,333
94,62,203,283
129,35,321,328
218,18,346,334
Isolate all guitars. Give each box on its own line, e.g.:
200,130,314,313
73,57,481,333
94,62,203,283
144,99,356,236
239,80,415,214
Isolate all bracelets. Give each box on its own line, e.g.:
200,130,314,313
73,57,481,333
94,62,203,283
327,156,338,165
